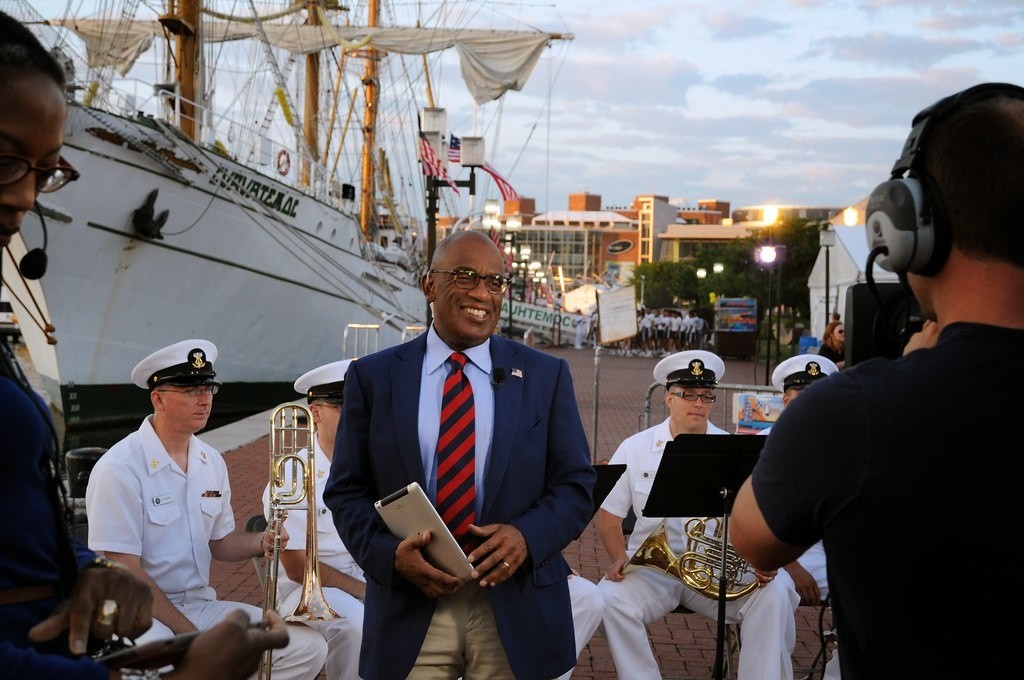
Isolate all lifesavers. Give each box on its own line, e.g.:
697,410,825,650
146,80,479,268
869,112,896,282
277,149,290,175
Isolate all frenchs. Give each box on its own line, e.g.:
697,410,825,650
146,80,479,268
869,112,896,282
620,515,759,601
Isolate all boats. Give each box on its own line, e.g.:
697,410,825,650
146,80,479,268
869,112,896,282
0,0,576,493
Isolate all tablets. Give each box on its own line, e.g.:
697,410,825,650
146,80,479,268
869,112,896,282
374,482,475,589
92,619,270,668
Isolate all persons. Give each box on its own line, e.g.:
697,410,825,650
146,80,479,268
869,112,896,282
574,309,585,349
726,84,1024,679
818,320,845,371
553,292,562,310
87,340,328,679
768,354,843,679
585,307,599,348
323,228,599,679
0,7,289,680
610,306,705,357
262,356,368,679
554,567,605,679
596,350,802,680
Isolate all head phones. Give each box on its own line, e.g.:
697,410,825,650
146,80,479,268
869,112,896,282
864,82,1024,276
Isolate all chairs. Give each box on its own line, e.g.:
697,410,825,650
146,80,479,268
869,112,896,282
624,504,835,679
244,514,271,603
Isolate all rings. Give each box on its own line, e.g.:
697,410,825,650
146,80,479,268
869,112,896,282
503,560,511,568
95,598,120,627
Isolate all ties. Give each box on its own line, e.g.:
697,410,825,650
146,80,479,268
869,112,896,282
435,352,475,562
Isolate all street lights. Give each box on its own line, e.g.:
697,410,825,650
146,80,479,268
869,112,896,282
757,246,777,386
818,220,838,328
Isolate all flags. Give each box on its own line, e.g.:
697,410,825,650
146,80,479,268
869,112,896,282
418,130,461,194
449,135,519,201
487,225,553,302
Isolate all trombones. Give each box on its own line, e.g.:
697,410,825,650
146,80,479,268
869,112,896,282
256,404,347,680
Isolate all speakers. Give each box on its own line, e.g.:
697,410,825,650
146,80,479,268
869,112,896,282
845,282,928,371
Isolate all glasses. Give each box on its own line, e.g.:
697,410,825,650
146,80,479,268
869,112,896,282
669,389,718,404
157,385,219,397
430,265,513,294
0,156,79,193
315,403,344,414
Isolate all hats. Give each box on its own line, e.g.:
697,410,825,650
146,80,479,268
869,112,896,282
293,358,354,403
129,339,222,390
653,350,725,389
771,354,838,390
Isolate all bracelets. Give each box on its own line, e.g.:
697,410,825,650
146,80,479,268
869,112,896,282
90,555,116,567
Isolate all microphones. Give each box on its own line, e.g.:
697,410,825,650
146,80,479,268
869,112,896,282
20,201,48,279
494,367,506,380
